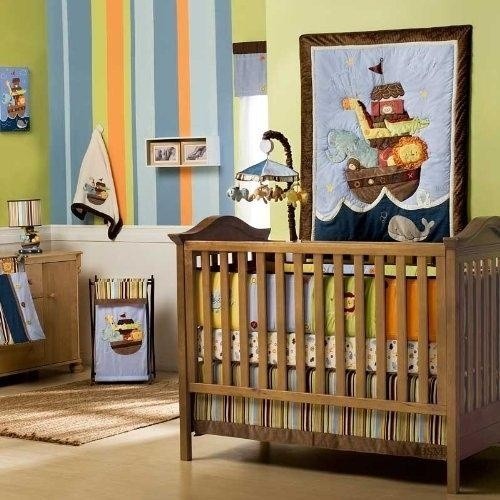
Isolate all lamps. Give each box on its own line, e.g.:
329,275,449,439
7,197,45,253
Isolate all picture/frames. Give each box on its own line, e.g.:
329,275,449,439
181,140,206,165
148,142,181,166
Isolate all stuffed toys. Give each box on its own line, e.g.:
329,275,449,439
226,182,309,208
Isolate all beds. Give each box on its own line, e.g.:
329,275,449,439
167,214,500,494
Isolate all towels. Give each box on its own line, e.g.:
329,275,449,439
70,126,124,243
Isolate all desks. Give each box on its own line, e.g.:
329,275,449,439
0,247,83,379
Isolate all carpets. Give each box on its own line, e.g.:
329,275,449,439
1,370,178,446
